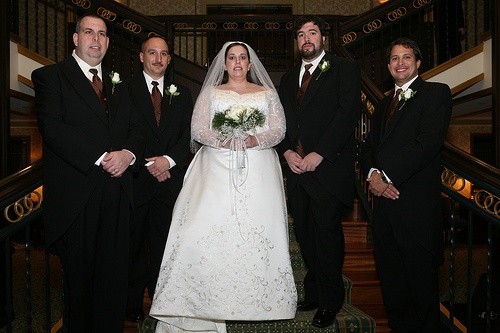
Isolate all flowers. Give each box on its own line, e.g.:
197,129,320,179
316,59,330,81
398,88,418,111
165,84,180,105
108,71,124,95
212,104,267,140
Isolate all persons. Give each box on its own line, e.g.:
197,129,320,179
278,18,359,326
150,40,297,322
360,38,452,333
30,15,193,333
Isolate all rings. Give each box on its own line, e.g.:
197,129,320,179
114,170,117,173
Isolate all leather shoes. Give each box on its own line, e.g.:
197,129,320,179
312,303,342,327
296,300,318,311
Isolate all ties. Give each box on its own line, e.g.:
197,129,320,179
295,64,313,159
382,89,403,132
89,69,106,111
151,81,162,128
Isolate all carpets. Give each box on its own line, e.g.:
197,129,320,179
138,212,377,333
